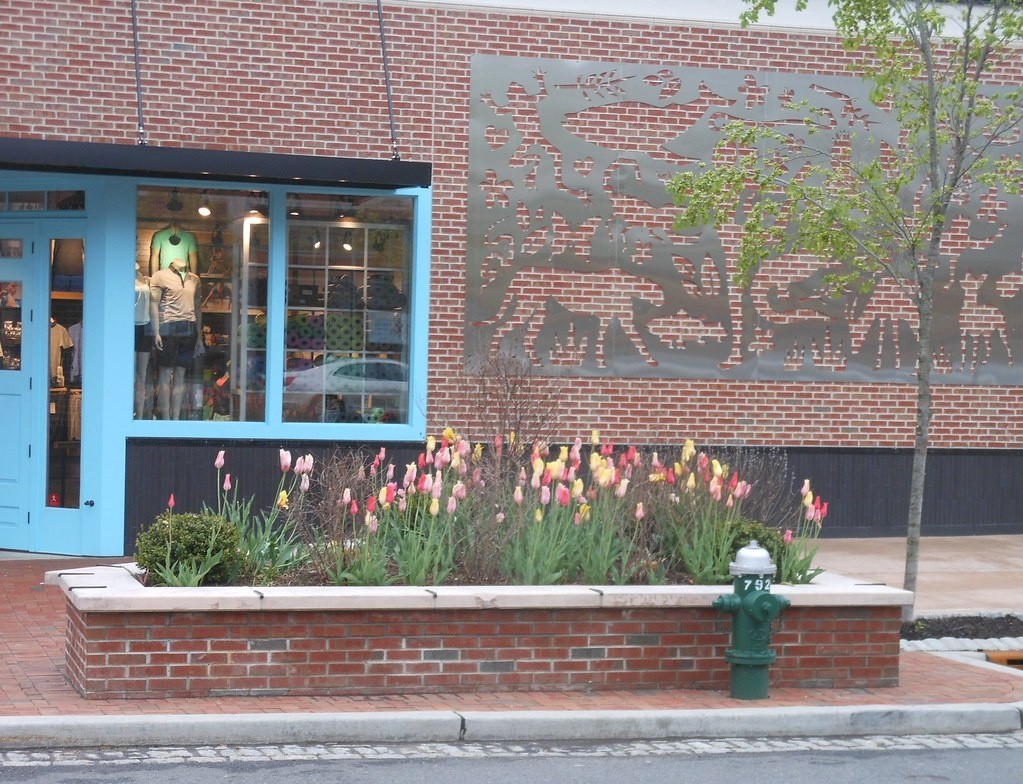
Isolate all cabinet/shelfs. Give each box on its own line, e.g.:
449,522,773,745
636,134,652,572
230,217,414,423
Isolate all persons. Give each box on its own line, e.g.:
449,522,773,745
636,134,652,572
151,226,198,274
0,281,19,308
203,247,232,309
135,263,151,419
150,258,203,419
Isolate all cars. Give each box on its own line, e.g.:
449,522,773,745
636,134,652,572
246,355,409,424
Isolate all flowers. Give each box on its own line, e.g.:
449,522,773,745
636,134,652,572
164,426,832,587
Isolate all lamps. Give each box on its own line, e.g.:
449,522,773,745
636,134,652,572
198,189,212,217
246,190,270,215
343,231,354,251
167,187,184,212
313,230,321,249
287,193,304,218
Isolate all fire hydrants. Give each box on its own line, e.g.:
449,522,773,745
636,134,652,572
711,538,792,700
